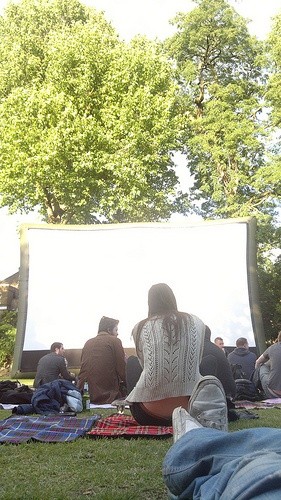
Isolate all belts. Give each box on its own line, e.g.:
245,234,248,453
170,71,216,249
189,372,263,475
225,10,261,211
139,403,172,421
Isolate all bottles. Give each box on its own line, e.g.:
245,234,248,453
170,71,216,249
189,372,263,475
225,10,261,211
82,381,90,412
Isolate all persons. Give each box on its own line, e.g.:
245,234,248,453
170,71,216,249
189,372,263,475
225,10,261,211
214,338,229,358
124,282,217,426
75,316,127,405
161,374,281,500
252,331,281,399
33,342,75,388
199,325,237,400
227,338,257,380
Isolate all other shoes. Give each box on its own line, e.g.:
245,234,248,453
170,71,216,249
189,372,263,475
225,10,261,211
188,375,229,433
172,406,203,444
